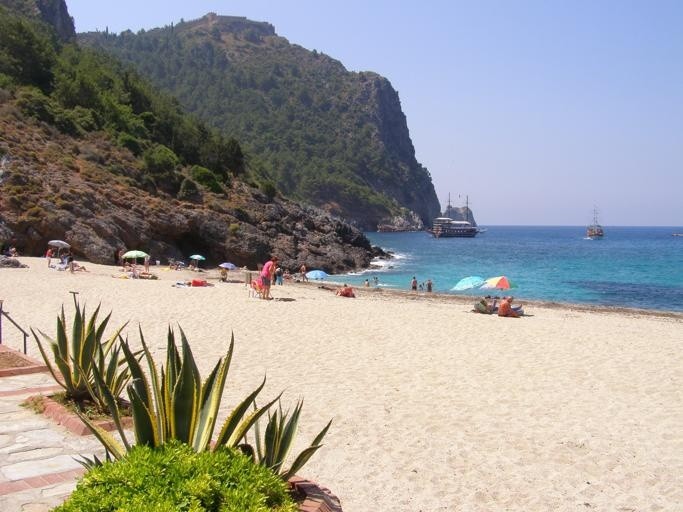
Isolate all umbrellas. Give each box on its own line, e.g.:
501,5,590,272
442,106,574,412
450,275,487,305
47,238,71,256
484,276,520,290
481,284,499,298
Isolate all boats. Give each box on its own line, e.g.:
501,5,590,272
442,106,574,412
670,232,682,236
375,224,409,233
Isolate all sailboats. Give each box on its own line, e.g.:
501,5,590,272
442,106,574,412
585,207,605,239
426,191,478,238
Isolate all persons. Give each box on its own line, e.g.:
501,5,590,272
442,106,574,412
245,255,309,298
317,283,356,297
167,257,203,272
365,276,378,287
497,295,520,318
2,247,20,257
425,279,433,290
480,297,523,316
113,248,159,279
218,268,228,282
410,276,417,289
42,248,90,272
